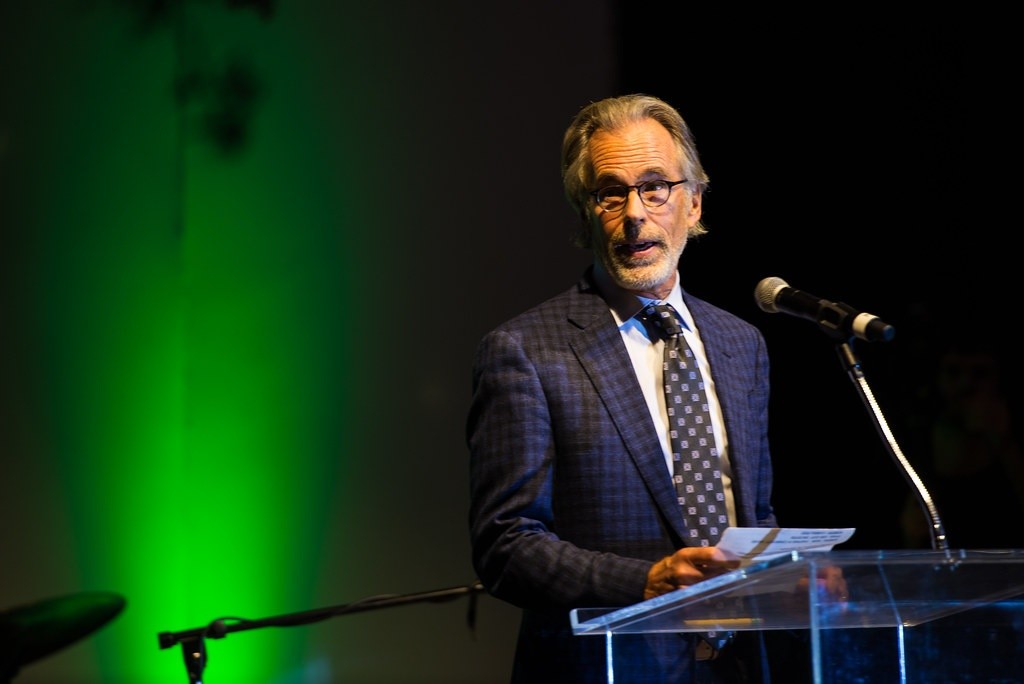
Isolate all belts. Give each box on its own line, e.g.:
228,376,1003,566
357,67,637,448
695,634,772,662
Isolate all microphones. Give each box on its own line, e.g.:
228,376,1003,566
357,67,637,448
755,277,893,345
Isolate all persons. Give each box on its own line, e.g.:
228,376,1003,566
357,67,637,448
869,485,985,684
465,93,848,684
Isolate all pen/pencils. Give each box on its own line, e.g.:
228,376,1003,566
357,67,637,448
684,618,765,629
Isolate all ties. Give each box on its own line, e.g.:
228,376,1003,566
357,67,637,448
632,303,745,651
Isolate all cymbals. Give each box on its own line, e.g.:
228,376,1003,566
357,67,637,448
0,589,128,684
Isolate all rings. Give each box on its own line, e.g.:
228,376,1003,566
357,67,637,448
840,594,848,600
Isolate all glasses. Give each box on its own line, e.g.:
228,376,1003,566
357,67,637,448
590,179,689,212
